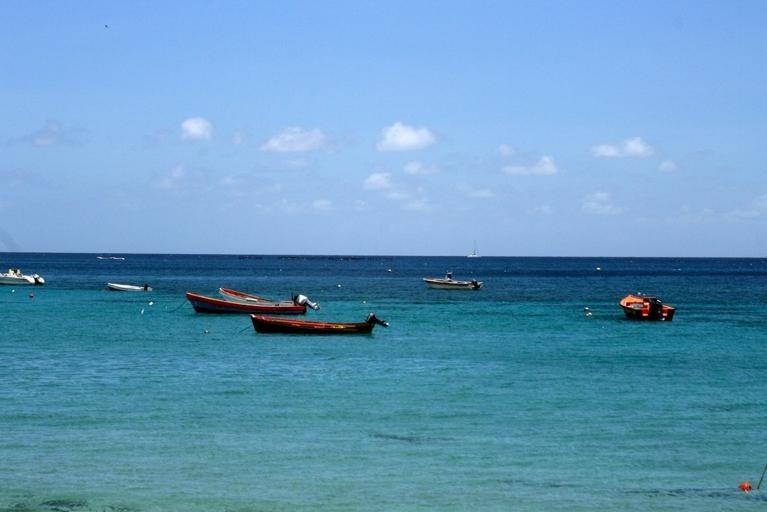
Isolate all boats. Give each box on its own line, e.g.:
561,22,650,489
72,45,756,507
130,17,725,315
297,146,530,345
422,277,484,291
107,283,152,292
248,312,389,335
185,292,307,315
217,287,272,303
619,294,676,321
0,269,46,285
